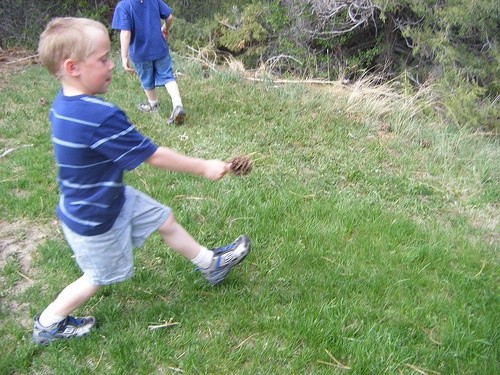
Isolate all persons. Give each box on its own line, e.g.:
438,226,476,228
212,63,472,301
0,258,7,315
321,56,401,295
112,0,187,128
31,15,253,347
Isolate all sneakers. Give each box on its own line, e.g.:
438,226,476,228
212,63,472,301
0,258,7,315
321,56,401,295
198,234,251,287
32,313,96,344
167,105,185,127
138,103,160,113
